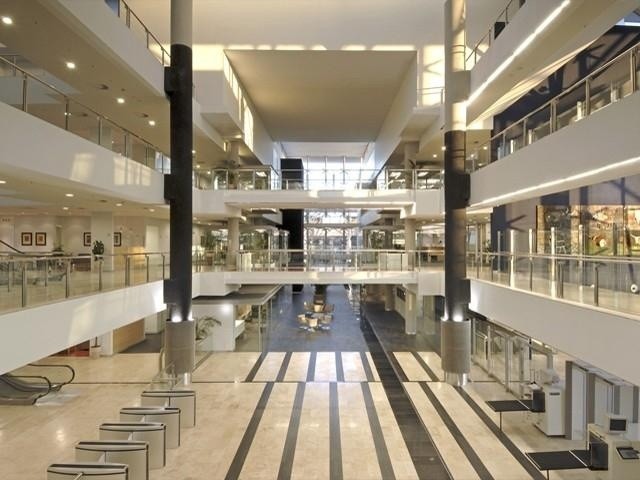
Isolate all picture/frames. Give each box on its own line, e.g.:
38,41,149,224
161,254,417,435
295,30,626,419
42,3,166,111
21,232,32,246
114,232,121,246
84,232,91,246
36,232,46,246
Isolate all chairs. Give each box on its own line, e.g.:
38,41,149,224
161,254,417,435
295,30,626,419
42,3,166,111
297,301,334,333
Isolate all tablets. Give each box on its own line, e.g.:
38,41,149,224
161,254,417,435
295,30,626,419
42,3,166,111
615,446,640,461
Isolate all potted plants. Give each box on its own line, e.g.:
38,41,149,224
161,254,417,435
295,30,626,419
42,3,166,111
205,229,216,265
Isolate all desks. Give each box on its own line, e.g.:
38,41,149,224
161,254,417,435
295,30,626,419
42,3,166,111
525,451,608,480
485,400,546,431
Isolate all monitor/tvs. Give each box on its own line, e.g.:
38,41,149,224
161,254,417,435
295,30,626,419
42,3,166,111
540,368,560,385
604,413,628,433
